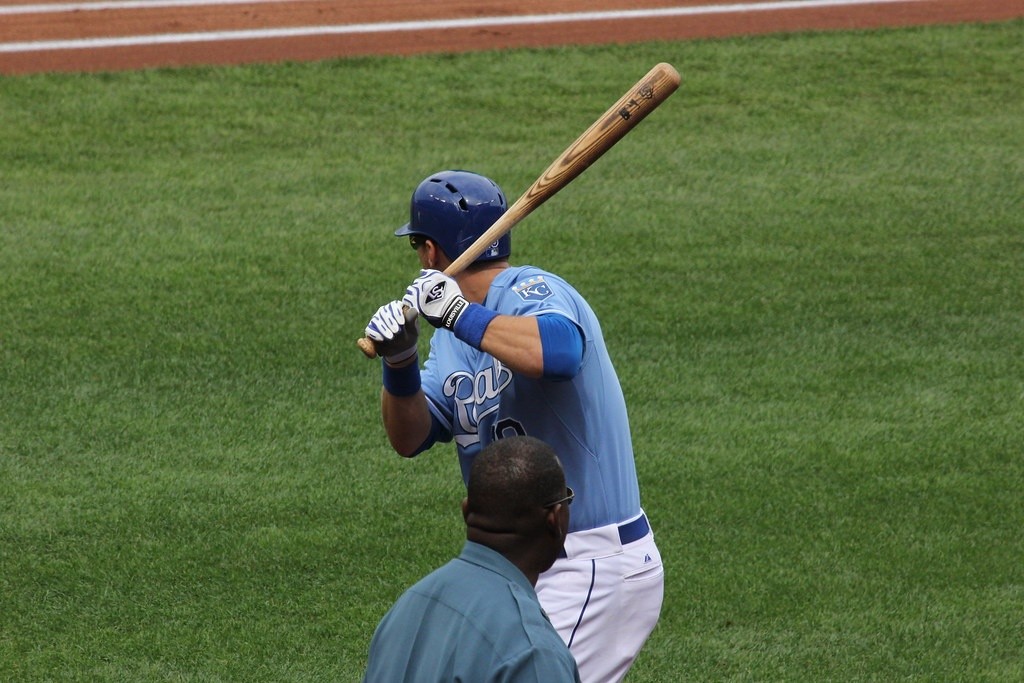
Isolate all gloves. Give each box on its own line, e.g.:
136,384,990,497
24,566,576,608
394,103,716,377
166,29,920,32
365,300,422,396
402,269,501,353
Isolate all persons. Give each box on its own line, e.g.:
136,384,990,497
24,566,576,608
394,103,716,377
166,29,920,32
368,435,583,682
364,170,664,683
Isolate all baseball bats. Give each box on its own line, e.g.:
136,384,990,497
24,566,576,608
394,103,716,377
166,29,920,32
353,61,685,351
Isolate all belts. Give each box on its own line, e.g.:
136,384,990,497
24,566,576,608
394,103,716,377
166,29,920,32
558,514,649,558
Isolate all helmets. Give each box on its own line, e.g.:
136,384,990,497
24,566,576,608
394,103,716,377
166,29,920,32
394,170,512,262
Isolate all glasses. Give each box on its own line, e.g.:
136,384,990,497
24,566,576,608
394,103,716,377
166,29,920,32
544,486,575,508
410,234,427,250
